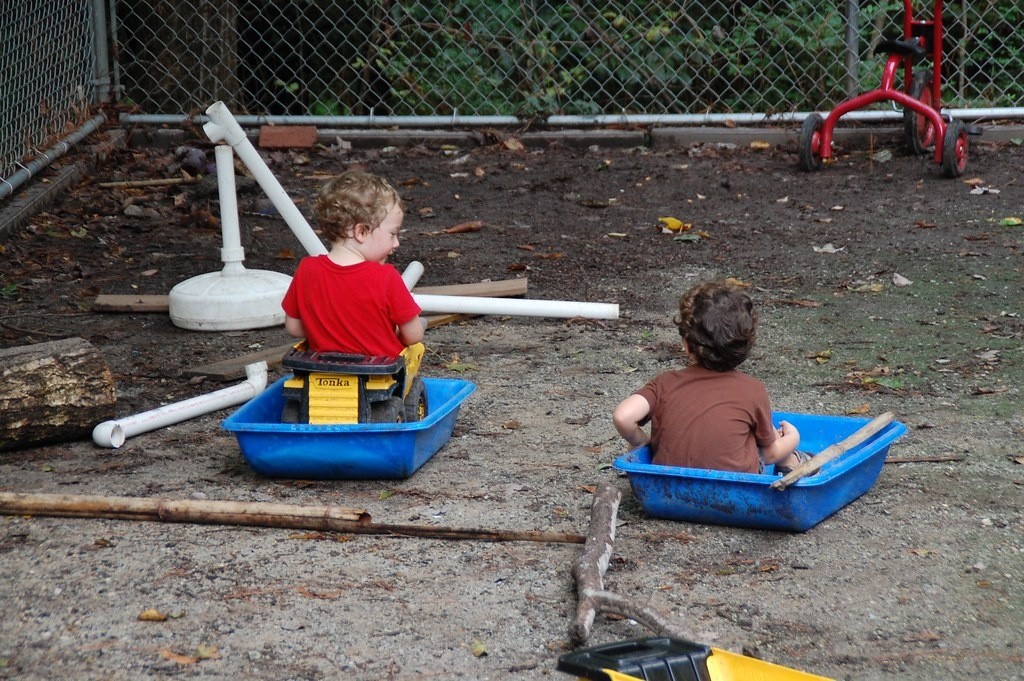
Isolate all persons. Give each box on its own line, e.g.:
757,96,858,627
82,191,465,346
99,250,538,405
612,282,822,479
281,169,424,359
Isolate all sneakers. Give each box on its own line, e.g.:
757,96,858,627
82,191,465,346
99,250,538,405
774,450,819,478
